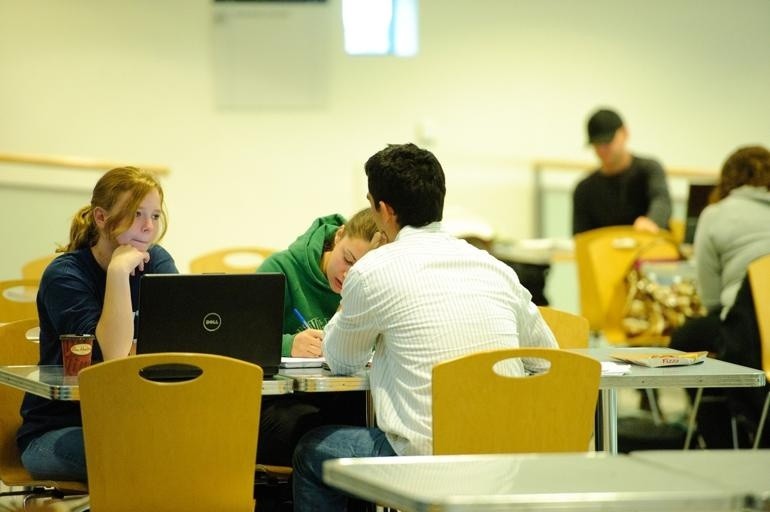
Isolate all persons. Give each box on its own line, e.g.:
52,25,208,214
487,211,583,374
17,167,180,479
255,208,386,463
573,110,672,237
670,147,769,450
292,144,560,510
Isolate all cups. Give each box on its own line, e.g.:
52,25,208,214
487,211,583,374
61,333,95,377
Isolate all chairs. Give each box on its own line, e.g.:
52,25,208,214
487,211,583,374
0,220,770,512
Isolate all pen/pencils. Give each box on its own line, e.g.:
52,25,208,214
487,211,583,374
293,307,311,329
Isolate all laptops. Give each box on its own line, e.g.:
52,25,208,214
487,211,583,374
136,272,285,378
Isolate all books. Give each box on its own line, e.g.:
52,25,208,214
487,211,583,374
281,357,327,369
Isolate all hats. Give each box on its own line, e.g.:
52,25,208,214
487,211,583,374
586,109,622,147
